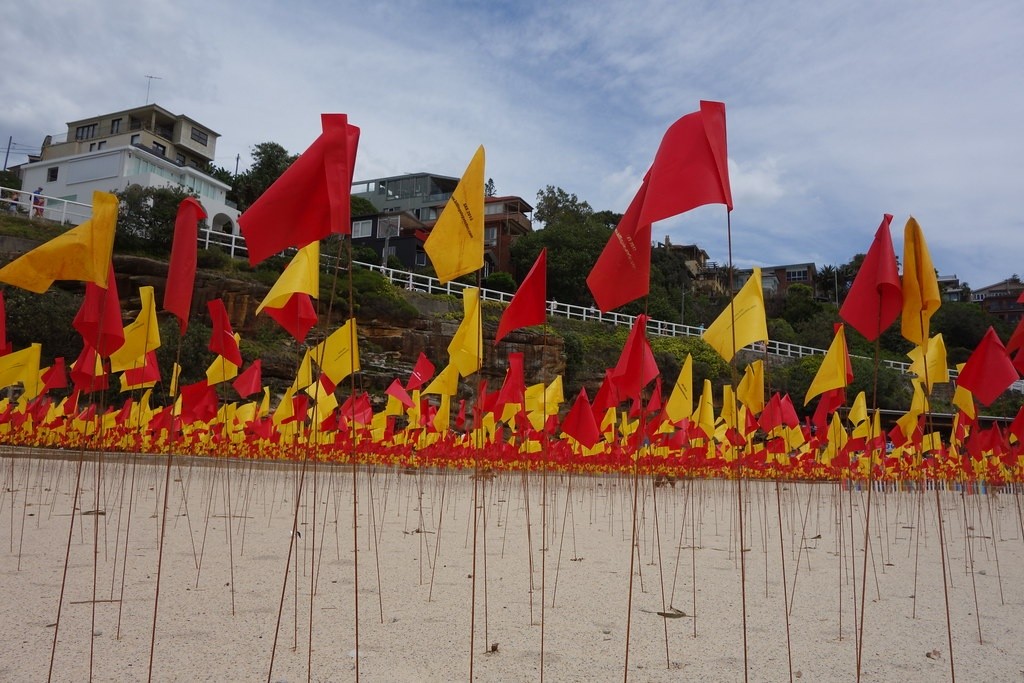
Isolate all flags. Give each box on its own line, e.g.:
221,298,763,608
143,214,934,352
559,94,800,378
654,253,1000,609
0,213,1024,499
0,191,120,294
423,144,486,286
236,113,361,345
585,100,734,314
163,197,209,337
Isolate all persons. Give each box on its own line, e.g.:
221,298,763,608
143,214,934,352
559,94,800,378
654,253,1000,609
9,192,19,215
29,187,44,218
